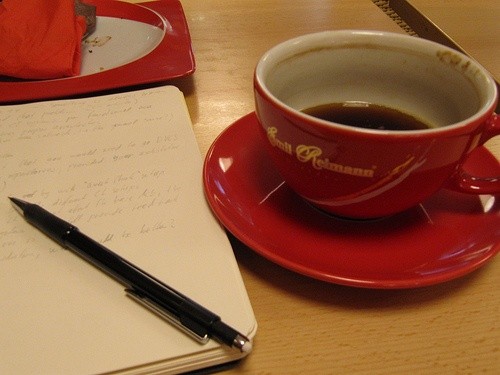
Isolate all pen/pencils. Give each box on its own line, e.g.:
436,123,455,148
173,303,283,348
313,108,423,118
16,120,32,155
8,196,252,354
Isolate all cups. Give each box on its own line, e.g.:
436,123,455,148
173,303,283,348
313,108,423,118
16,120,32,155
253,29,500,221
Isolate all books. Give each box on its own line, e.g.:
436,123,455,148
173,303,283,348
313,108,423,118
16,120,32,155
0,85,257,375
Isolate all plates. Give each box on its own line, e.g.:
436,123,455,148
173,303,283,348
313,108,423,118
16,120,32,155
0,0,197,105
204,110,500,291
